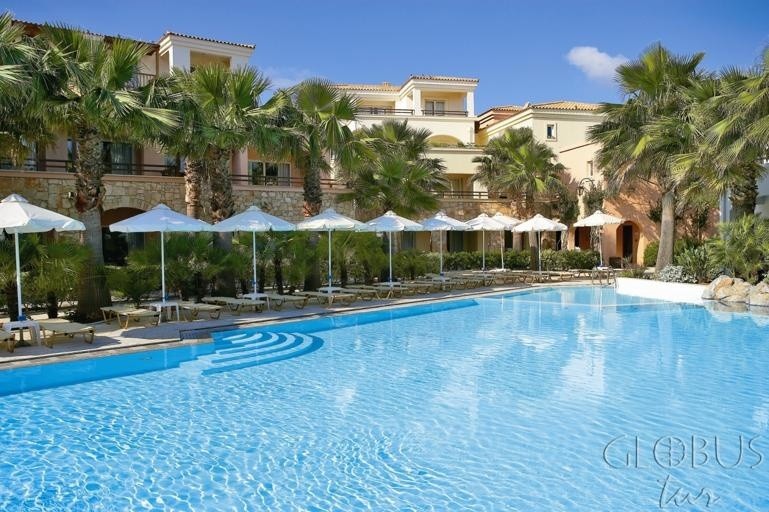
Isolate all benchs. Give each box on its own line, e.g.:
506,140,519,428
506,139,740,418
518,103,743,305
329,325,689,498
0,266,621,353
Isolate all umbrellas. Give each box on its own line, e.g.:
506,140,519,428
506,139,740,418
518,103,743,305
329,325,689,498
572,209,626,266
354,210,428,282
212,204,298,294
108,202,218,308
0,193,87,342
407,210,471,275
489,212,525,269
510,212,568,282
461,212,506,271
296,207,374,291
